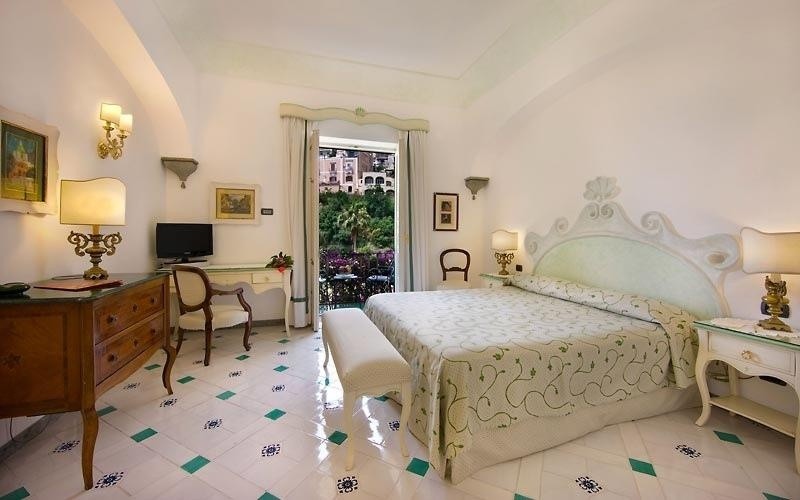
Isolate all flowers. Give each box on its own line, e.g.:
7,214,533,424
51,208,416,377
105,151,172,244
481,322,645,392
265,250,294,274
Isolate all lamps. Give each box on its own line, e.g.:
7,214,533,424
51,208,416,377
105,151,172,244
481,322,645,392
741,225,800,333
58,176,128,280
96,102,135,161
490,228,520,275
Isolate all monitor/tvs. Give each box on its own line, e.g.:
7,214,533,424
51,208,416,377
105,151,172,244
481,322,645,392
156,223,213,264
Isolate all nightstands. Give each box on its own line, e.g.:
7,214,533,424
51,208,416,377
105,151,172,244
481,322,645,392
686,316,800,477
478,272,514,288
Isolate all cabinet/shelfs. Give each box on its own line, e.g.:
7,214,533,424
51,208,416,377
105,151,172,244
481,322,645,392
1,270,178,492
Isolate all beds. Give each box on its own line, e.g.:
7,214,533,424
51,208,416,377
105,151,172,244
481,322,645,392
364,174,741,489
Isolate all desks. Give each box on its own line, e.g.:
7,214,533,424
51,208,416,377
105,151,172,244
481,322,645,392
155,264,293,339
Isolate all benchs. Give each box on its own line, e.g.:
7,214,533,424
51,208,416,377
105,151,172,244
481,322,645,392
318,307,415,472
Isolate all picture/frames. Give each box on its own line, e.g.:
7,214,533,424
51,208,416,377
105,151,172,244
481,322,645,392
432,191,460,233
208,181,262,227
1,103,62,218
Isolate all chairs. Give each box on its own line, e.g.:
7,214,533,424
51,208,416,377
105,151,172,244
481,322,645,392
169,264,254,366
437,247,472,292
